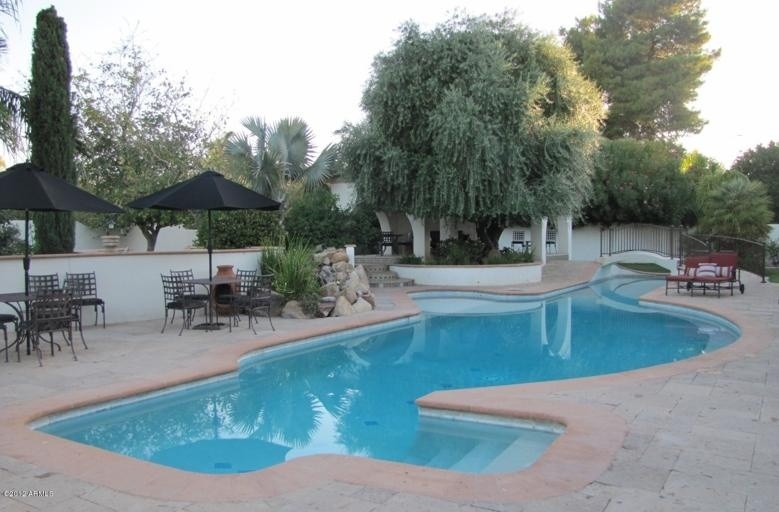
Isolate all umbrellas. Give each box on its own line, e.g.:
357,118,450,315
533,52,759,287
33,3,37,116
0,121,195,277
0,160,125,355
126,170,281,323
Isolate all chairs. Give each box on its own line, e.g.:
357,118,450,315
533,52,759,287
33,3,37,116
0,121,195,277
158,268,277,337
663,248,746,298
509,229,557,255
0,269,107,367
376,228,413,254
